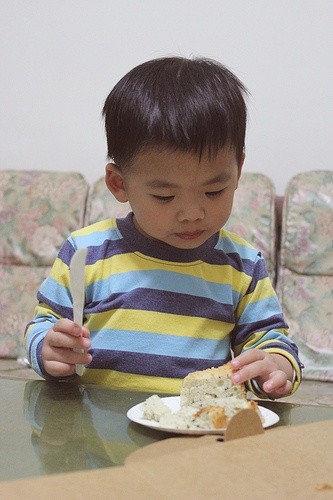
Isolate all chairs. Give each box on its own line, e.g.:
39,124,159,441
1,166,333,408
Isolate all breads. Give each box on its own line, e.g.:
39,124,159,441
143,394,171,421
178,363,246,407
160,397,265,430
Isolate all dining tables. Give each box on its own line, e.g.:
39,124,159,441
1,375,333,500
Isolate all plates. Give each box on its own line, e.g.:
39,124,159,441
127,396,280,434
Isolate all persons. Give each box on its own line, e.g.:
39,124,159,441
23,56,305,401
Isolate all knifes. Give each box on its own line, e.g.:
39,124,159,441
67,247,89,377
